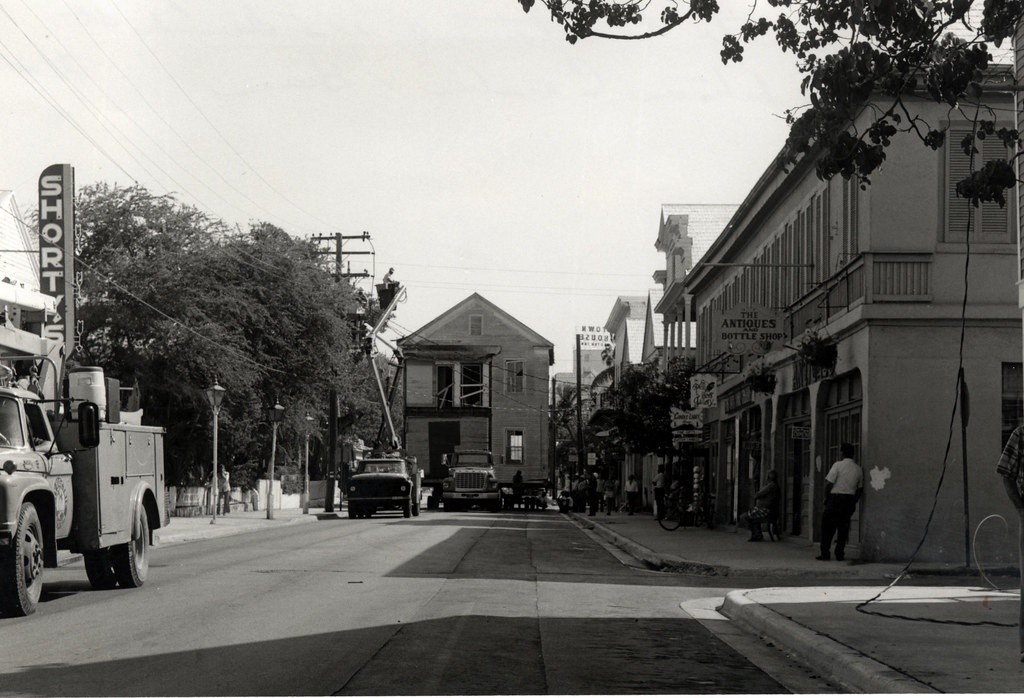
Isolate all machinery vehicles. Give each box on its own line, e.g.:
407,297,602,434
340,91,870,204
339,281,425,519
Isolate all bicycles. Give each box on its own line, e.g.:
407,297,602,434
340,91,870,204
656,481,704,532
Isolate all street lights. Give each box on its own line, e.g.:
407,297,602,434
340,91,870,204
266,394,286,520
204,374,228,525
301,410,315,514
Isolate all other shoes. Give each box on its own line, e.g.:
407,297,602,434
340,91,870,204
816,555,829,561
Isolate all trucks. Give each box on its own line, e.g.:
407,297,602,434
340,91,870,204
440,449,503,513
0,356,167,617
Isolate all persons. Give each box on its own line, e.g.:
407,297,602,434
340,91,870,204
217,464,231,516
652,466,665,520
816,442,863,561
383,267,395,289
557,472,615,516
752,467,760,490
624,474,639,516
535,492,548,510
512,470,523,509
747,470,780,542
